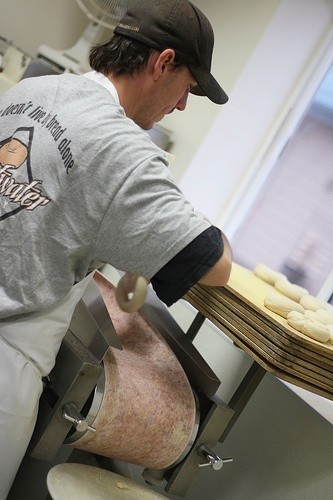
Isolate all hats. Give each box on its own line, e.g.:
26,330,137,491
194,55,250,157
113,0,229,105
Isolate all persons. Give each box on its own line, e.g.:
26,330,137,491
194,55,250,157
0,1,234,500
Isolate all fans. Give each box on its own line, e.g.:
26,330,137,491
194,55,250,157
36,0,140,77
